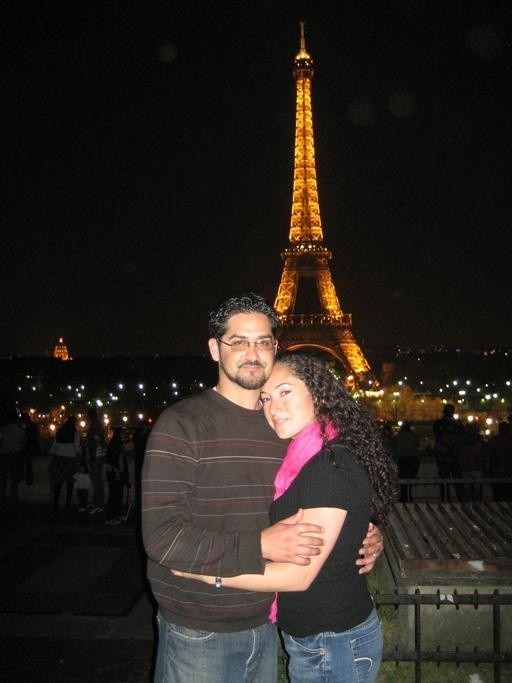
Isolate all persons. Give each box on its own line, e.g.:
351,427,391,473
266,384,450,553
386,403,510,501
172,351,402,680
1,404,150,526
141,291,385,681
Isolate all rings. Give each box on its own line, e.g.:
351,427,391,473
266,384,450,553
372,553,377,560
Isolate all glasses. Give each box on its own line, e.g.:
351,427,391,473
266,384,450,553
218,338,275,352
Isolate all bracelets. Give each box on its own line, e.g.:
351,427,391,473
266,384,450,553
216,575,222,587
